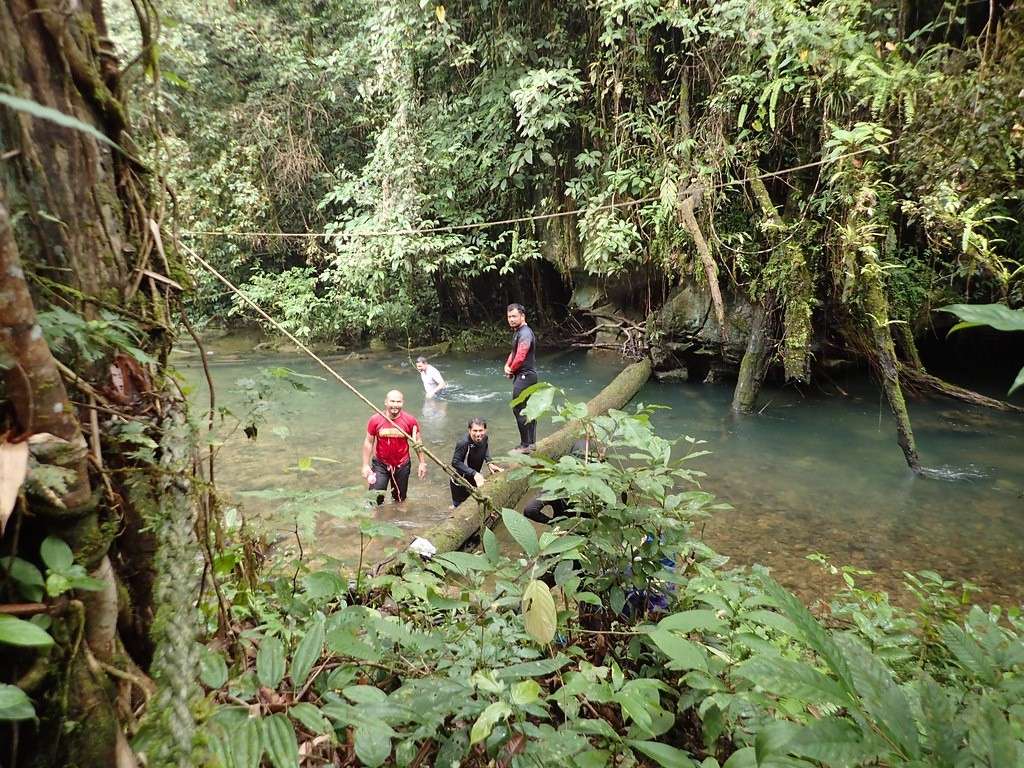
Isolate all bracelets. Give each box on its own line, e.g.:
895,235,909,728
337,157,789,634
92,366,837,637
419,463,427,467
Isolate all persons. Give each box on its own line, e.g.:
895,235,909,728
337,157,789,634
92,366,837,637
449,418,504,509
416,356,447,400
504,303,538,454
502,438,676,643
362,390,427,507
